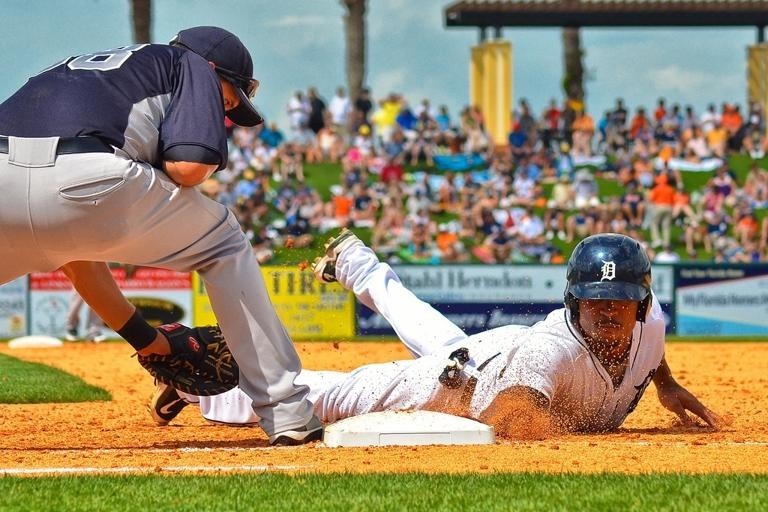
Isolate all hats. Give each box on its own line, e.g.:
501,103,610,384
566,231,649,303
169,25,265,128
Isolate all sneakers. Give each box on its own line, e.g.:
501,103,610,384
312,226,358,284
268,413,322,447
150,383,189,424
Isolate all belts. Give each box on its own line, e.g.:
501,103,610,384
462,352,498,408
2,137,113,154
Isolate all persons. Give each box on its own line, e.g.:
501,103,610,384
66,294,108,342
197,82,768,266
0,24,321,446
147,225,723,439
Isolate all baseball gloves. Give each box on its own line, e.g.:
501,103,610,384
138,323,240,394
